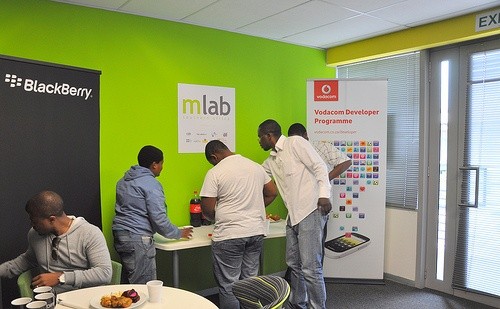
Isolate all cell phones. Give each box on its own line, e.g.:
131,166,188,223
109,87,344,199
324,232,371,259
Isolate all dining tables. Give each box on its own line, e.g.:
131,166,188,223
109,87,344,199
47,284,219,309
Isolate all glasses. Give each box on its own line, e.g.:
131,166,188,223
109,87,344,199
51,237,61,260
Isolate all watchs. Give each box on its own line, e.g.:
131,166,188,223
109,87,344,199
60,274,65,285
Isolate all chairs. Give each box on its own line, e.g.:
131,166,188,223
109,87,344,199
17,257,122,298
232,275,291,309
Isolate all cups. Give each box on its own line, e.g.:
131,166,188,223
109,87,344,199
33,286,52,298
183,226,193,239
266,219,270,234
35,293,55,309
11,298,32,309
26,301,47,309
147,280,163,303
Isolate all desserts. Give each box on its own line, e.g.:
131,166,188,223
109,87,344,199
121,289,140,303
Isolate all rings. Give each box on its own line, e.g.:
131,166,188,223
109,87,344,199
37,285,39,288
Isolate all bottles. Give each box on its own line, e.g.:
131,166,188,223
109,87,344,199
190,191,202,227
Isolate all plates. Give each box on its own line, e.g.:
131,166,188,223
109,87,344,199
271,218,282,223
90,290,146,309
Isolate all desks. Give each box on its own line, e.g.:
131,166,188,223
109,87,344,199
153,217,287,288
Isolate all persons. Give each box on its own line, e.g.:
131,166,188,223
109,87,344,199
285,123,351,282
199,140,278,309
258,120,332,309
0,191,112,295
112,146,193,284
